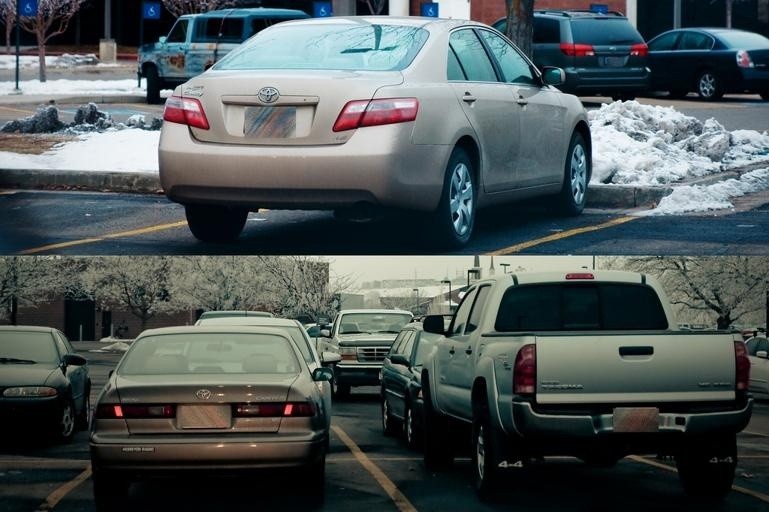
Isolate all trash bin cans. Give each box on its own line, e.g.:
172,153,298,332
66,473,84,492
99,39,117,63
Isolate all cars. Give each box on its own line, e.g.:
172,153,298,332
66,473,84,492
156,13,595,248
679,320,769,399
183,305,370,442
0,324,95,448
371,313,461,453
643,24,769,104
82,323,338,501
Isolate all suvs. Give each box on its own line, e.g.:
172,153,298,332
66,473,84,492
133,4,318,105
488,8,654,103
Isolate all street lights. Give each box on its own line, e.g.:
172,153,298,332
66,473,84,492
500,263,512,273
439,279,453,312
466,267,479,288
413,287,419,311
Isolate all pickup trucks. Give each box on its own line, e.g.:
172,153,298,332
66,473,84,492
420,266,756,493
315,306,420,398
